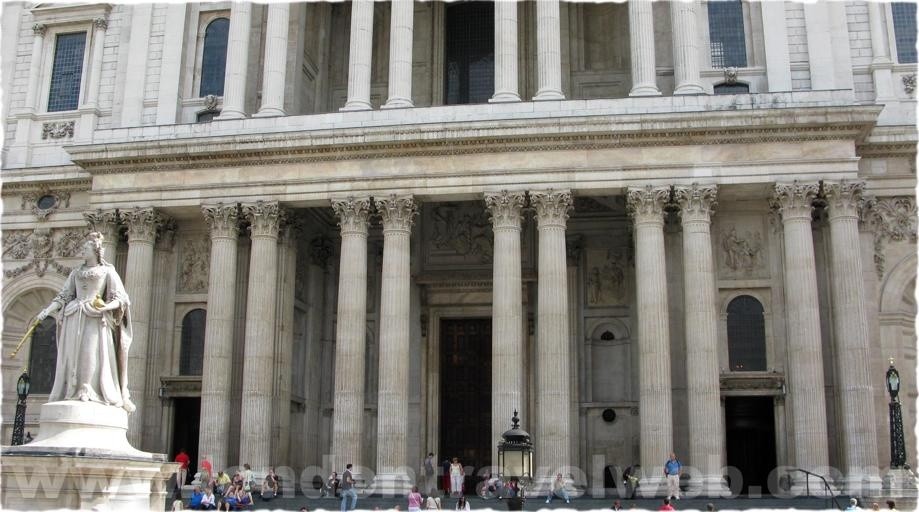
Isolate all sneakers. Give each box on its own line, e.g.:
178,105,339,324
565,500,570,503
676,496,680,501
666,496,671,501
545,499,551,504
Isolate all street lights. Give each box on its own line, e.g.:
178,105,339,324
9,370,33,446
881,356,912,472
491,409,542,512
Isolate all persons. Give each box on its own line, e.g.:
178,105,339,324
589,248,627,306
872,502,880,511
657,498,674,511
38,232,138,413
664,452,683,501
706,502,715,512
179,234,211,293
886,499,899,511
171,445,637,510
845,497,861,511
723,227,764,281
